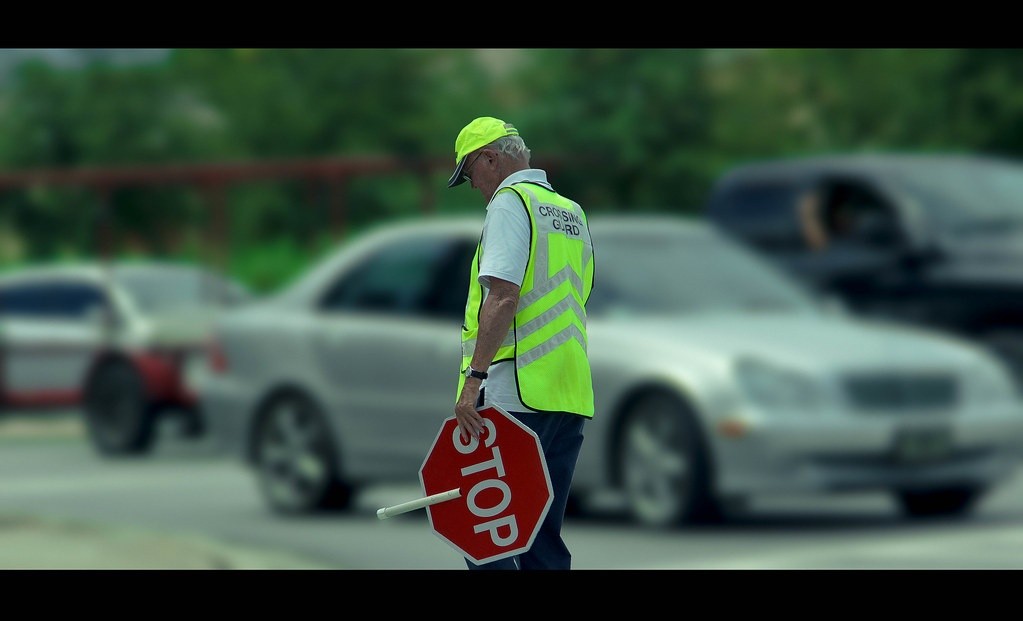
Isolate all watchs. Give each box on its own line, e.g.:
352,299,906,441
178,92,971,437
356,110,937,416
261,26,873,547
465,365,488,379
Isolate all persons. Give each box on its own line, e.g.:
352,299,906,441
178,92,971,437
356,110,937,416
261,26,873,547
446,116,596,569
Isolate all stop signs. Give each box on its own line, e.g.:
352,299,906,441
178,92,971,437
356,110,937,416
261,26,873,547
416,403,556,566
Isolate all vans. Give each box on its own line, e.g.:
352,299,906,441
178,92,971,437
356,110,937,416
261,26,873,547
707,152,1022,344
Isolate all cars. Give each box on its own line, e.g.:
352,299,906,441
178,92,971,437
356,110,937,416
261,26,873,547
169,206,1023,531
0,251,254,455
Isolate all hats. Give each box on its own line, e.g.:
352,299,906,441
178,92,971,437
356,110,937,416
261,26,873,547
447,117,519,188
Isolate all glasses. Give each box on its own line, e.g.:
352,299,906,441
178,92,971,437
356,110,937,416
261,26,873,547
463,150,498,183
